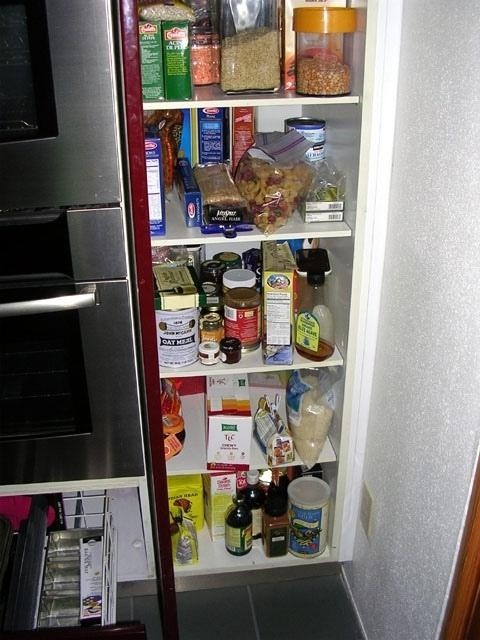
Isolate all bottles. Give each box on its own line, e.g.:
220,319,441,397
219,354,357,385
294,261,339,362
219,1,357,98
224,469,289,558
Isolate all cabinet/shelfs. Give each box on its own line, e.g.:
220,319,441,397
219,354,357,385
138,82,371,579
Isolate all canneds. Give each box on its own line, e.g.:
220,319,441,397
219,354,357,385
199,250,263,366
189,26,221,86
285,115,327,172
